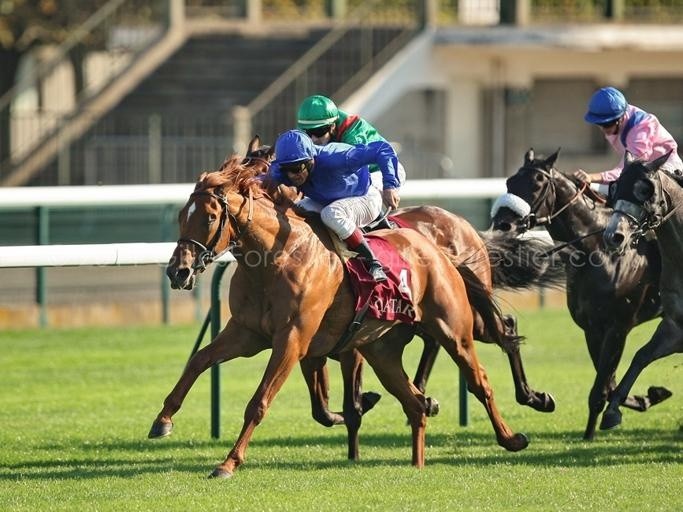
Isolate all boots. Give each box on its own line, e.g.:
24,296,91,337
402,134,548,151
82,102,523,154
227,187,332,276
347,237,389,283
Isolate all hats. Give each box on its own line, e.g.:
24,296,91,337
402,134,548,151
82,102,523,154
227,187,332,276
271,128,318,165
583,87,629,124
296,93,340,132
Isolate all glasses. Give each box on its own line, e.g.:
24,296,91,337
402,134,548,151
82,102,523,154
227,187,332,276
277,160,308,174
305,123,334,137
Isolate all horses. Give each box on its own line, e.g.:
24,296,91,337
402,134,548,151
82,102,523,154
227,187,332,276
147,152,530,481
490,145,683,444
598,147,683,430
239,134,585,462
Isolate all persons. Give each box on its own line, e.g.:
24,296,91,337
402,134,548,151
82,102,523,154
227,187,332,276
260,130,401,282
571,86,683,195
294,95,406,196
